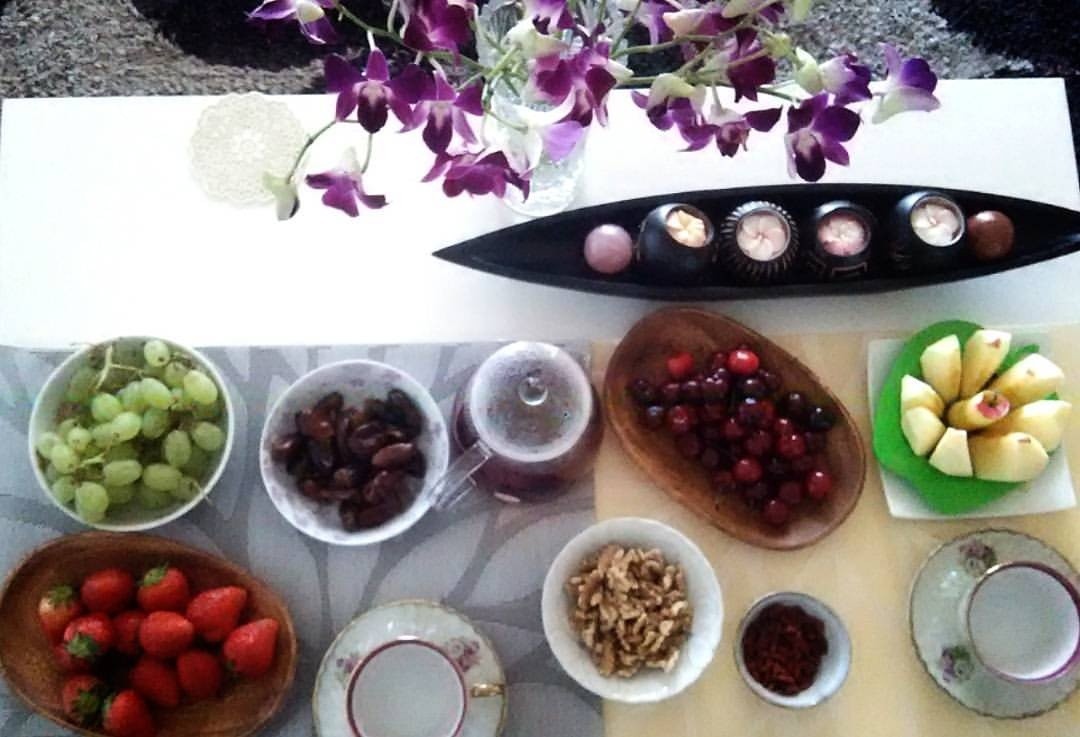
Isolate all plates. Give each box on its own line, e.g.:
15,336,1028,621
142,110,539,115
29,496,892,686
863,330,1078,521
432,183,1080,302
311,595,508,736
600,305,867,552
0,530,299,736
908,528,1080,720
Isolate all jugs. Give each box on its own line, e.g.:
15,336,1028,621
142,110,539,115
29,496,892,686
429,341,604,515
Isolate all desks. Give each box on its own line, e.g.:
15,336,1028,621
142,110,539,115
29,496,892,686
0,78,1079,737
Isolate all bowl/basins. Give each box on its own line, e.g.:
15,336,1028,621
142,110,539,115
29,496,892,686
256,359,450,545
24,338,234,531
541,518,723,702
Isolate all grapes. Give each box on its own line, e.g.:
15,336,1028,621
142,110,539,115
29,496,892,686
35,338,225,524
626,348,841,528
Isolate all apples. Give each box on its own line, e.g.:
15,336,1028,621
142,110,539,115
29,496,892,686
899,328,1069,483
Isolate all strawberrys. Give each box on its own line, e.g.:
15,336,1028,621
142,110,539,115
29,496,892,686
38,561,281,737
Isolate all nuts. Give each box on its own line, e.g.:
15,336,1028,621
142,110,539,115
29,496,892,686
567,544,692,677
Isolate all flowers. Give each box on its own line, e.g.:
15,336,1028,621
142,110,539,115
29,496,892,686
248,0,942,221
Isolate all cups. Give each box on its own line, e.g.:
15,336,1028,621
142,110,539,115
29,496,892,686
189,94,313,206
720,200,803,287
959,563,1080,686
627,204,720,286
804,197,883,284
734,590,849,709
891,191,970,264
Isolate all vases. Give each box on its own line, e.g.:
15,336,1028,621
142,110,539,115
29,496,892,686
476,0,592,218
